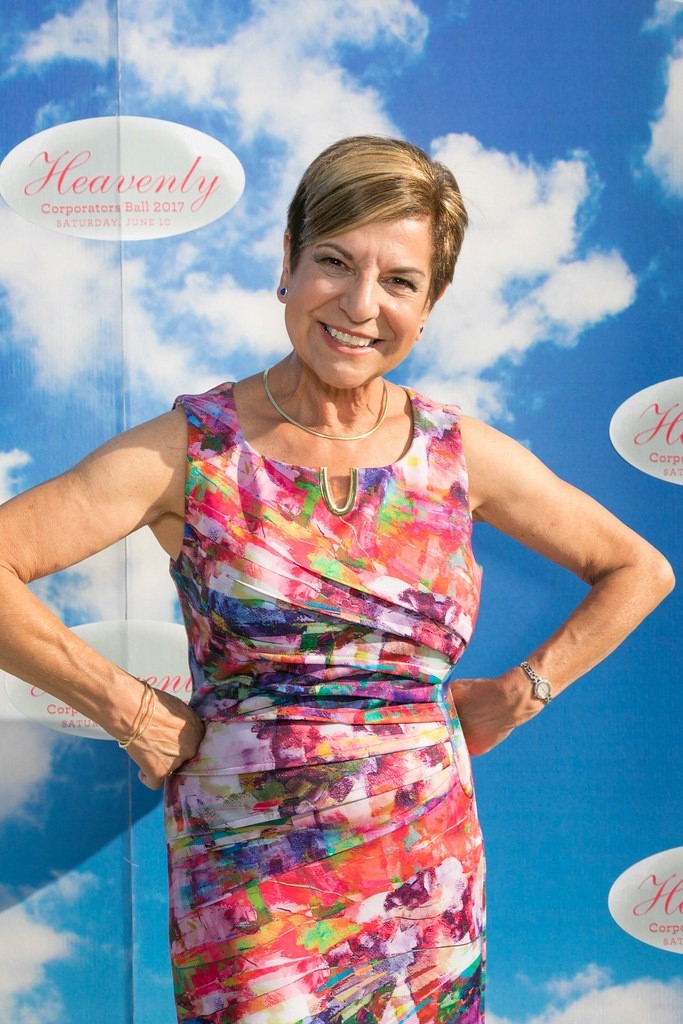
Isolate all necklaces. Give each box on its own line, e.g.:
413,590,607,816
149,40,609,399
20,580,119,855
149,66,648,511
263,366,388,440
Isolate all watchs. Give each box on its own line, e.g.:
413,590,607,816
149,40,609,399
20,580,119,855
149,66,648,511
519,660,553,704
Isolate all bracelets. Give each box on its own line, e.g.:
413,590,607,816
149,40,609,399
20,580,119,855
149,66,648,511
116,678,155,750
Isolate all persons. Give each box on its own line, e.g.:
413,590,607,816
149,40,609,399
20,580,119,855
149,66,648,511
0,135,676,1024
